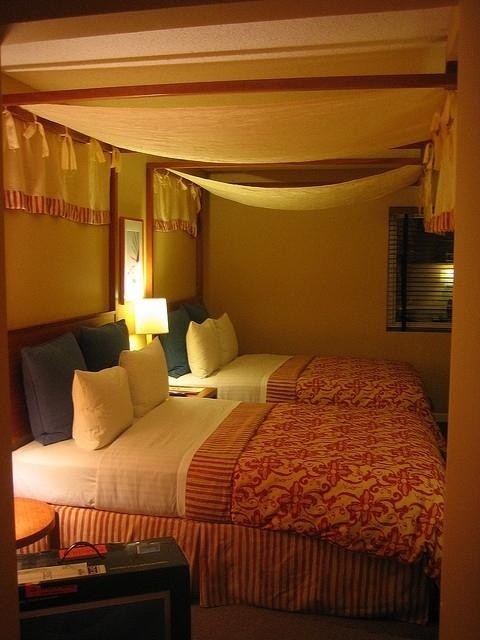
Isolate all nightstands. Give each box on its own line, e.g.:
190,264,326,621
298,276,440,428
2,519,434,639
169,385,217,399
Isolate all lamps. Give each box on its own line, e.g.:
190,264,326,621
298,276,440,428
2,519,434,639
134,298,170,345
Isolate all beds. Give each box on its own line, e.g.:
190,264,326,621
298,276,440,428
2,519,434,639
154,292,429,408
8,310,447,627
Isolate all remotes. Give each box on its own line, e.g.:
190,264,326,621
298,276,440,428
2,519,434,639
169,392,186,397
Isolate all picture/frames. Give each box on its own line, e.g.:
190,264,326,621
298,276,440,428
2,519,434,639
119,216,146,305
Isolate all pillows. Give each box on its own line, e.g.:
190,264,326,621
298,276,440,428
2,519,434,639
119,335,169,418
20,332,88,446
184,302,209,323
158,309,192,378
71,366,134,450
207,311,239,365
185,319,223,378
77,319,129,370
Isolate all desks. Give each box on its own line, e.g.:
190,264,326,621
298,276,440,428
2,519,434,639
14,498,60,553
